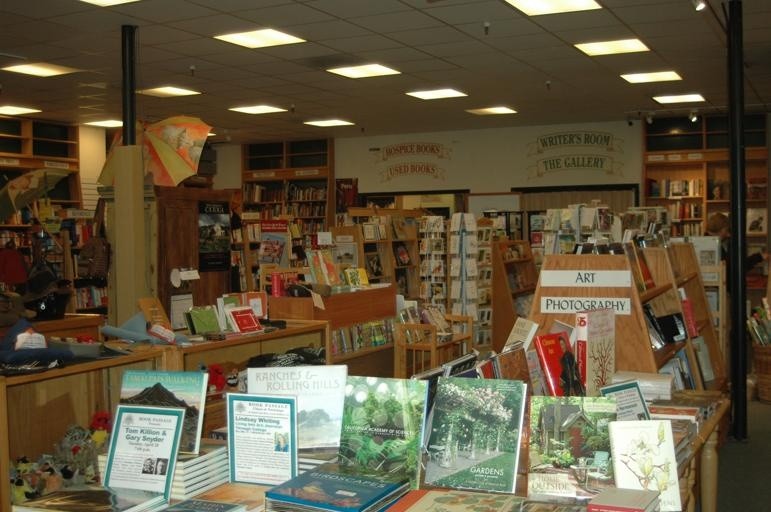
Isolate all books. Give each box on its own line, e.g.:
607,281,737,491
1,168,771,512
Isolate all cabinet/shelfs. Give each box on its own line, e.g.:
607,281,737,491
526,236,729,393
270,280,474,378
0,92,770,346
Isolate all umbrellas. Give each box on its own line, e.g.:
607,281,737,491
95,121,154,187
144,116,217,186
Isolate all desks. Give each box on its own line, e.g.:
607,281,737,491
1,359,732,512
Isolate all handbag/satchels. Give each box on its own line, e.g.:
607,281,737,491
78,237,108,281
27,259,57,290
